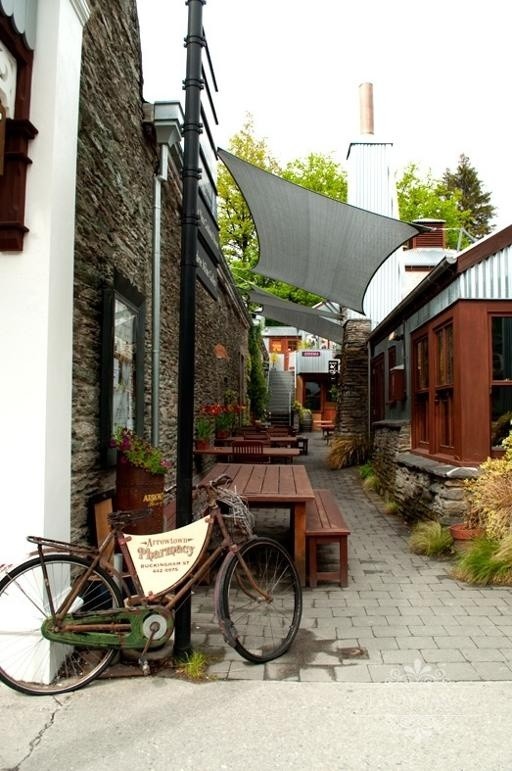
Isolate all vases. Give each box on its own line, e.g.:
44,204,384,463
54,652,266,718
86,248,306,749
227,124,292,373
115,450,165,573
450,523,483,561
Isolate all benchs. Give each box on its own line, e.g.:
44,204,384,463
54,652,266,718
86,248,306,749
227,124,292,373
304,488,351,588
164,480,207,532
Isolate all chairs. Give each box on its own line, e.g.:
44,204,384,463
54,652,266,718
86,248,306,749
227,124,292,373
192,424,309,466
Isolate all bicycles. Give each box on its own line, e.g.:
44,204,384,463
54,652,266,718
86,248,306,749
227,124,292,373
0,473,303,696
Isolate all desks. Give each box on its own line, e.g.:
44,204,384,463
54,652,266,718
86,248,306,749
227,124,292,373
199,462,316,588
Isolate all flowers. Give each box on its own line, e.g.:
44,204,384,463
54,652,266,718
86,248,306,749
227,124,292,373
109,421,169,475
195,405,231,439
